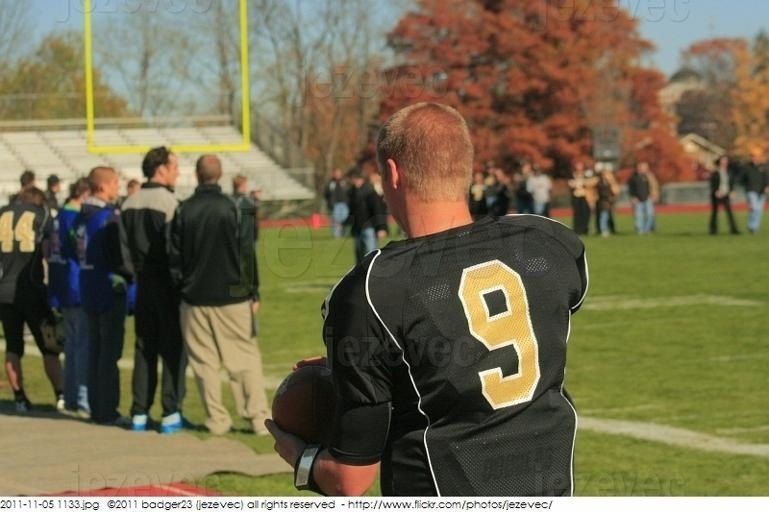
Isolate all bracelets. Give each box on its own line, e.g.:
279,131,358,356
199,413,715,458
293,442,324,496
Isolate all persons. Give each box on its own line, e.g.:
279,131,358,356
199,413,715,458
0,145,274,436
264,102,591,497
324,170,389,264
708,154,740,234
736,148,769,234
468,159,661,238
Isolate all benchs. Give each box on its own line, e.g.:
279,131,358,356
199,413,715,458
0,113,321,212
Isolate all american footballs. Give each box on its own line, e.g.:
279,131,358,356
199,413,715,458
273,366,337,448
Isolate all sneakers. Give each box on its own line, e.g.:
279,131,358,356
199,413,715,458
159,411,197,434
132,415,152,430
